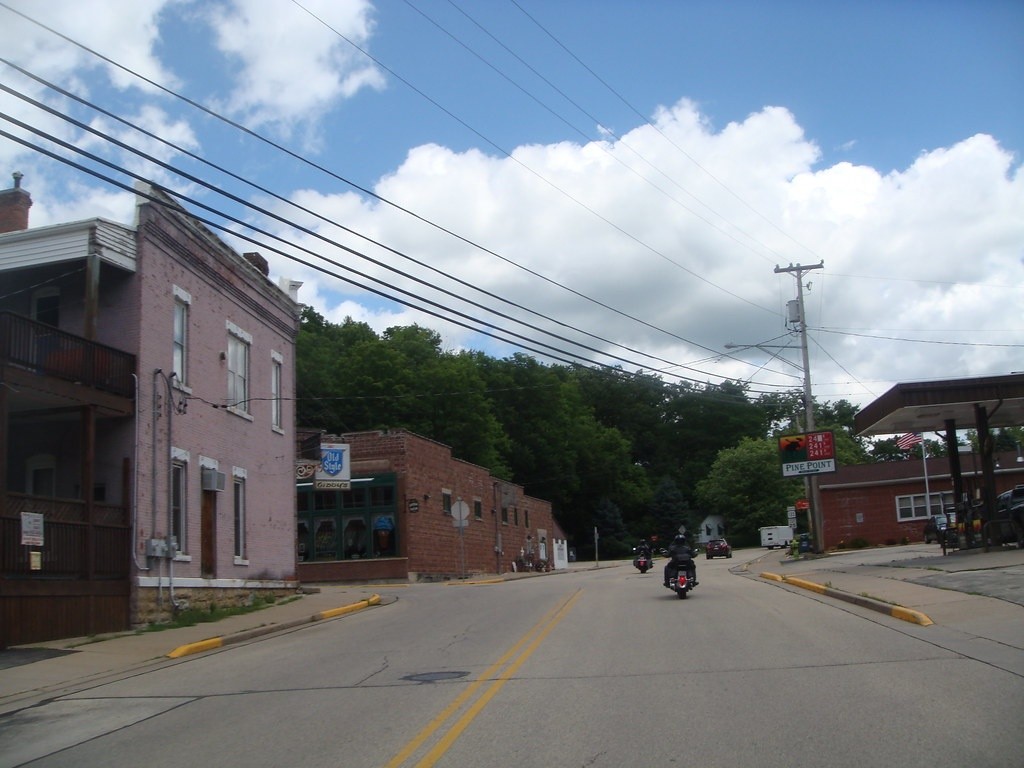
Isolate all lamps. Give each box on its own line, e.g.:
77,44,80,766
491,509,497,513
424,494,433,501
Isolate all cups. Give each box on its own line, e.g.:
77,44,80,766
378,530,390,549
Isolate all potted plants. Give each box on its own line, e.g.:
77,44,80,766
516,556,551,572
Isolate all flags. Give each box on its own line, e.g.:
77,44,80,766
896,431,922,452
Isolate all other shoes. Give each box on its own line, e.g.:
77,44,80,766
693,581,699,586
663,582,670,588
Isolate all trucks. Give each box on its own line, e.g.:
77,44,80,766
758,526,794,550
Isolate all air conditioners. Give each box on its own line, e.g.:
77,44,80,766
201,469,226,491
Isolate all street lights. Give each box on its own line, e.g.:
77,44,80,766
724,342,824,553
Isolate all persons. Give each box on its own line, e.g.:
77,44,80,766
662,534,699,587
633,539,653,568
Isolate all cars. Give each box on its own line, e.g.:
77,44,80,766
923,485,1024,544
706,539,732,559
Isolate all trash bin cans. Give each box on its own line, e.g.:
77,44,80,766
799,534,809,552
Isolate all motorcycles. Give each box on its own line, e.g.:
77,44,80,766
632,547,656,573
659,548,700,599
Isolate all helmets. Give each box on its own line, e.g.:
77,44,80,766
640,539,646,543
675,534,686,545
